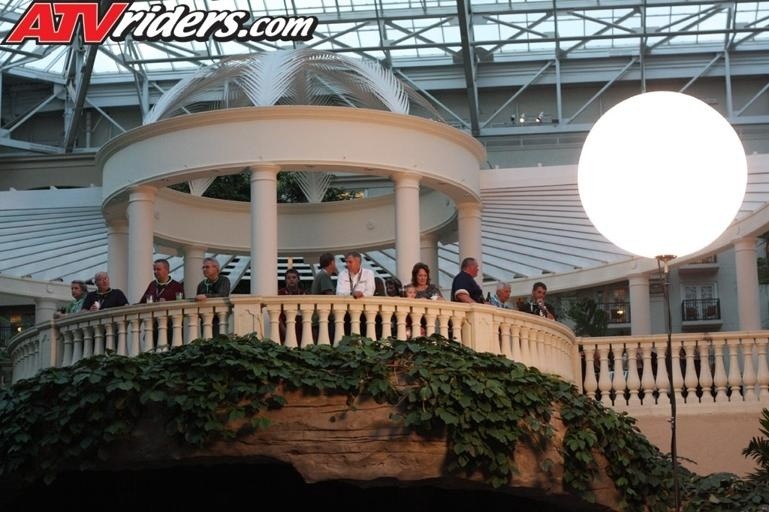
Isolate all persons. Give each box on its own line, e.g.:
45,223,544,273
193,258,230,337
308,252,336,345
78,271,129,353
489,281,514,309
451,257,484,303
335,250,376,338
276,268,311,346
134,258,184,353
53,280,89,318
520,281,556,322
372,262,450,343
